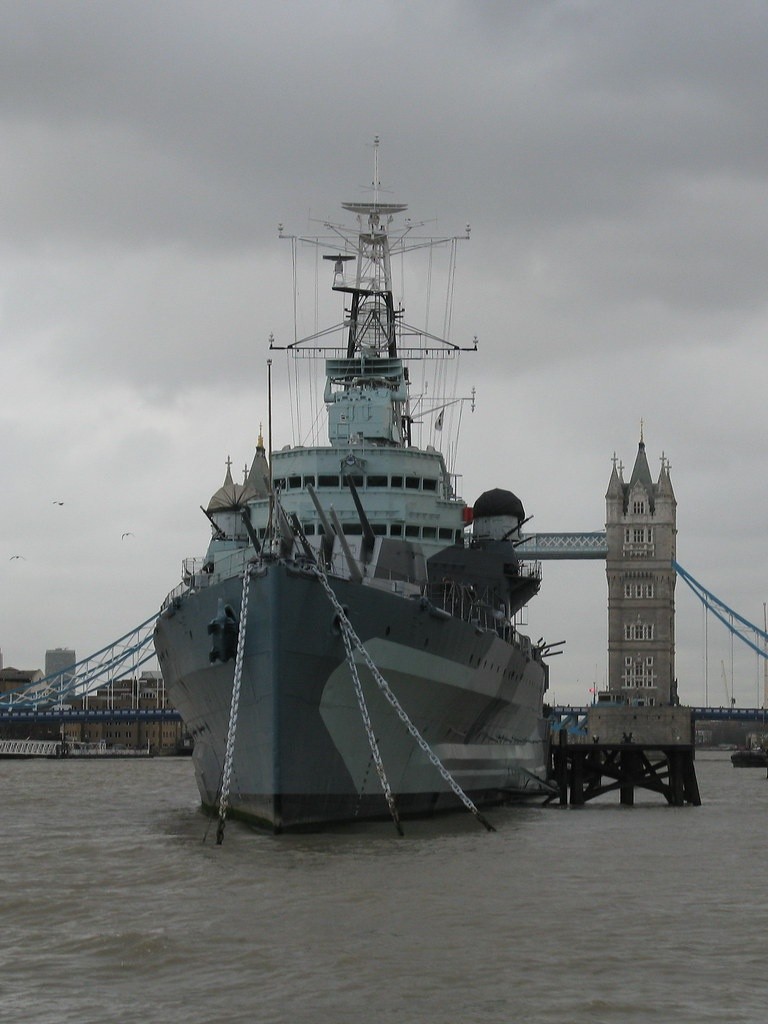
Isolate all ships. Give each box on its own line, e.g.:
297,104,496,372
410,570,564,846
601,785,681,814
149,128,568,838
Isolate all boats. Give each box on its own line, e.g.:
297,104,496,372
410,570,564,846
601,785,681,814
731,742,768,769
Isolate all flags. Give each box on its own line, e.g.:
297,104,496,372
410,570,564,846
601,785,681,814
435,411,444,431
